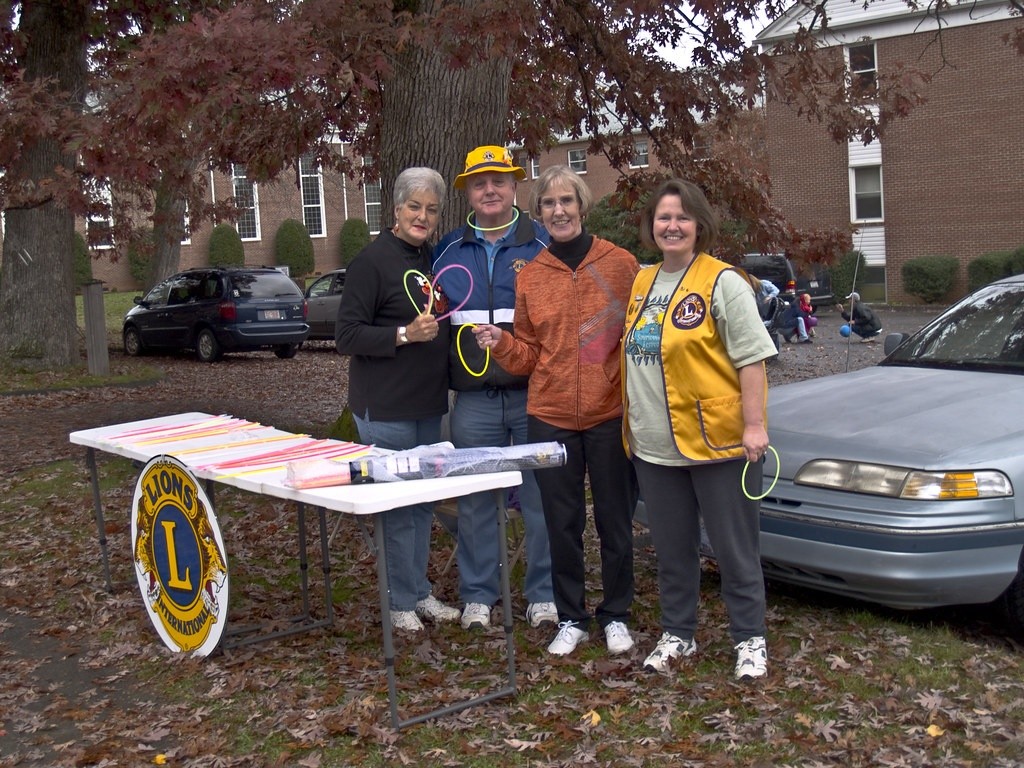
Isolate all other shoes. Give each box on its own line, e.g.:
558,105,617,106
783,333,791,343
799,339,813,343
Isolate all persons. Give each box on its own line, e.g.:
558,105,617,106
836,292,882,343
430,146,559,629
747,274,817,344
472,164,642,656
336,168,462,630
620,179,779,679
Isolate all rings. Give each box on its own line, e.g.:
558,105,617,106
763,451,767,455
479,340,483,344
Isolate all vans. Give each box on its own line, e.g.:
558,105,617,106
122,265,311,363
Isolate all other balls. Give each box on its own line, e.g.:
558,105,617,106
809,317,818,327
840,325,852,337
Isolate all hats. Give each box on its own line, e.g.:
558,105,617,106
845,292,860,301
454,145,528,188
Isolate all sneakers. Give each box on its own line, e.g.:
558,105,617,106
526,601,559,627
643,631,698,678
416,590,462,623
735,636,769,681
604,620,635,655
461,602,491,629
547,620,590,657
861,336,875,343
389,610,425,633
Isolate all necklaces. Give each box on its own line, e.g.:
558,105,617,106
467,206,520,231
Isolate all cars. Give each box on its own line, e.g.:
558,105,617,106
739,252,795,306
299,268,349,349
633,274,1024,640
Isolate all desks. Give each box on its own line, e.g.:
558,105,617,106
68,411,525,734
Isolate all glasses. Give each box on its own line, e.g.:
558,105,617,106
538,198,580,208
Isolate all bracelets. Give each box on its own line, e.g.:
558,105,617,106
399,326,411,343
